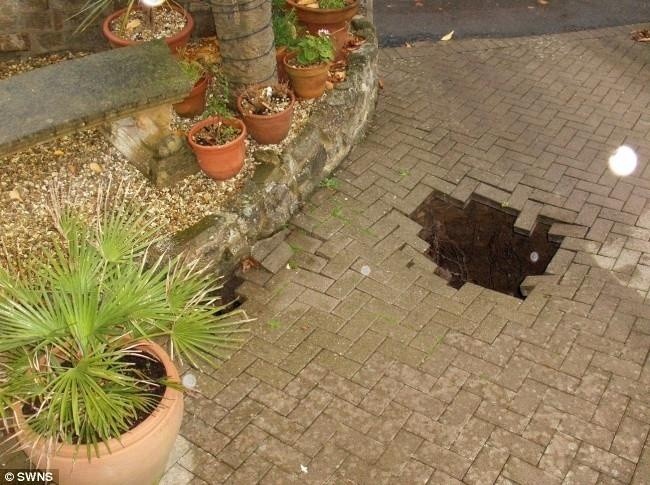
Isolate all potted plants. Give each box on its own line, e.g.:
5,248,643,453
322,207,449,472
97,0,363,182
0,167,254,478
